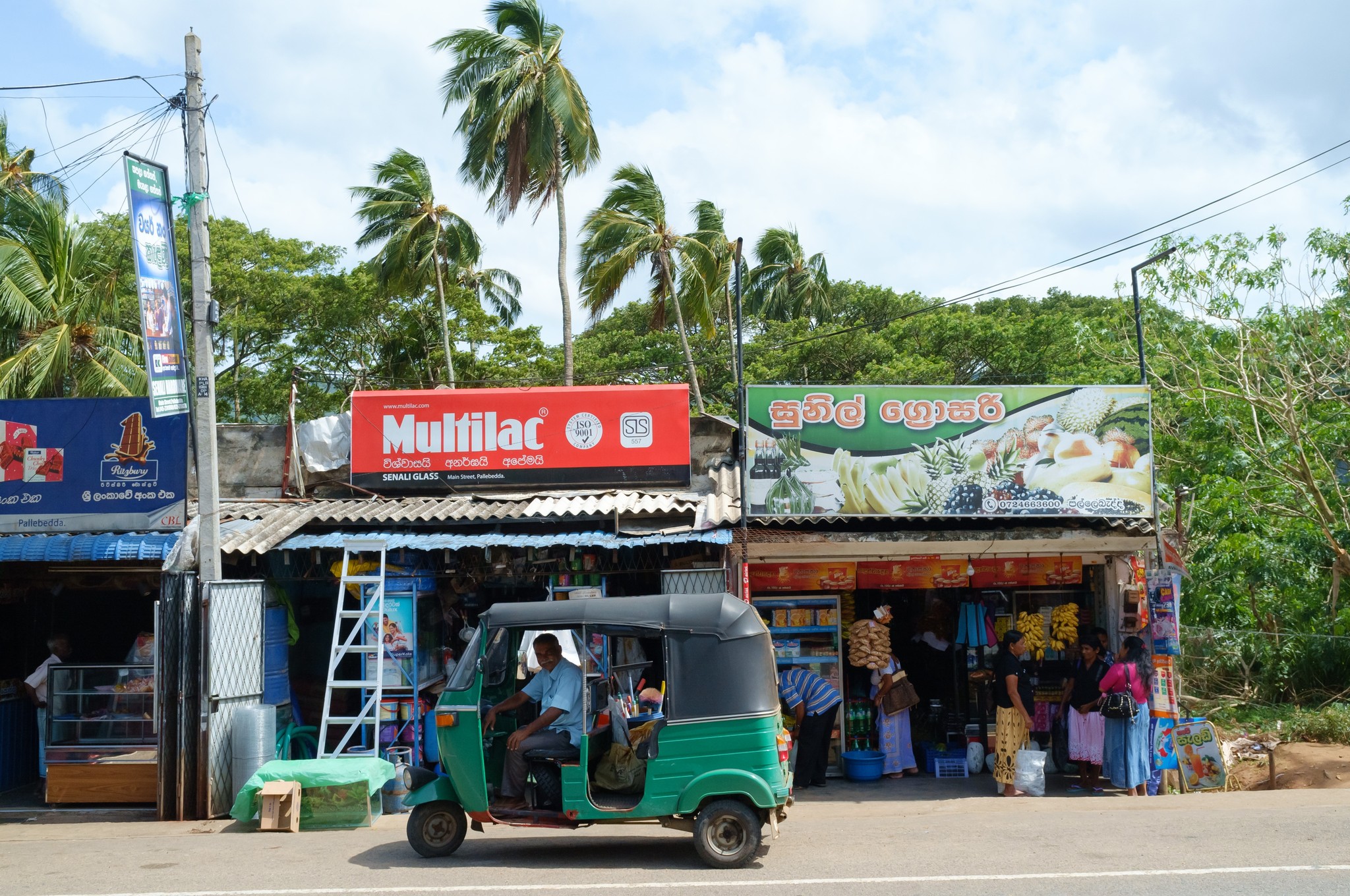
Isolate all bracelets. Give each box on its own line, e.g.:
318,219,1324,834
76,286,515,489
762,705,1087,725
877,693,883,696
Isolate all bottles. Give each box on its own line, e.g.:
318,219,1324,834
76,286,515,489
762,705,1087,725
549,554,601,586
851,736,861,751
844,699,855,735
855,698,866,734
865,697,872,731
863,735,872,751
810,646,838,656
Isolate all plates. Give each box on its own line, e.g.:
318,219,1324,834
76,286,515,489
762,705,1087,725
1203,761,1221,777
94,685,114,691
112,713,134,717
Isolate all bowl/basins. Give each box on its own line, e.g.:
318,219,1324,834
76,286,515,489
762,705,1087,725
841,751,887,782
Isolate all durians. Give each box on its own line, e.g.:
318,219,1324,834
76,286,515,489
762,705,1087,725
1110,397,1149,414
1057,387,1117,434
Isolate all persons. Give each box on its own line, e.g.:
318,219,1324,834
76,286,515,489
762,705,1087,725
870,653,918,778
1148,575,1172,591
374,614,408,651
1056,637,1109,795
1099,636,1155,796
777,669,843,789
24,634,72,797
482,633,595,816
1152,614,1175,638
993,629,1034,797
827,609,831,618
1077,626,1116,776
147,287,179,339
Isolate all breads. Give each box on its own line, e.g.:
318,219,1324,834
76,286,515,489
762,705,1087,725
143,709,153,719
111,676,154,693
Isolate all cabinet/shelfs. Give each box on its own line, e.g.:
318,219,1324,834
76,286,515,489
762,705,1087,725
361,582,448,768
549,578,608,680
752,594,845,779
43,664,158,803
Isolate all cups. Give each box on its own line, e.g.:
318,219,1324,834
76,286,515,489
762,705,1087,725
1056,577,1062,584
1064,576,1073,584
1071,574,1079,583
1042,571,1055,584
1190,754,1205,780
930,573,969,588
816,576,855,590
1047,574,1058,585
1073,570,1081,581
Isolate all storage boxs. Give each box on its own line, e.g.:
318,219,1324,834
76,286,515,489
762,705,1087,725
773,639,800,658
771,608,838,627
256,780,301,833
300,780,383,829
927,747,969,779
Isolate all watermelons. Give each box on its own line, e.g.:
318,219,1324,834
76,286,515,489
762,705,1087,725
935,743,946,752
1094,403,1150,457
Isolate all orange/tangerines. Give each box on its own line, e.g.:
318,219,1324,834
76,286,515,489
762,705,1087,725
1190,773,1199,785
1184,744,1193,755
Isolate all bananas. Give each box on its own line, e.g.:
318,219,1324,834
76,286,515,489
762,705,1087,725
1048,603,1079,651
831,447,865,514
1016,612,1048,661
863,453,931,515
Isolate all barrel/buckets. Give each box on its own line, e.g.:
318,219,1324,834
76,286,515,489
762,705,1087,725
373,698,398,721
373,722,424,762
627,711,664,730
276,703,293,761
264,604,290,706
399,697,422,721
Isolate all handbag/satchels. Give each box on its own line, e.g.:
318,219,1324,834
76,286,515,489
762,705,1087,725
978,669,997,715
1013,728,1047,797
1098,663,1140,724
877,658,919,716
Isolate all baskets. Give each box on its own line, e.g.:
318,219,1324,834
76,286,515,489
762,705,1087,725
922,741,968,772
934,758,969,778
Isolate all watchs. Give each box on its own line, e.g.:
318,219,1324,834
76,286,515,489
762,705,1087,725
796,725,801,728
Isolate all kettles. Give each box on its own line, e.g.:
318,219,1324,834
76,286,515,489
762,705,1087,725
249,573,278,607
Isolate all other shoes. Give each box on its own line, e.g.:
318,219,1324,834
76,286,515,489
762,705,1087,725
815,783,827,787
792,785,803,789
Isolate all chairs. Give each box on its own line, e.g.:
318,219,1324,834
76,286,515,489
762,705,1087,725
525,749,576,763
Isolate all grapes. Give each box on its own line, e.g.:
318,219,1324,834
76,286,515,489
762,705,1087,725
941,483,1064,514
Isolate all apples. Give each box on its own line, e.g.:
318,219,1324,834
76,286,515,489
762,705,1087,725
868,455,898,476
1038,432,1150,475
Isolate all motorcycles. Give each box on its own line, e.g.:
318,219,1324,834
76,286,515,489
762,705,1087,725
400,592,793,870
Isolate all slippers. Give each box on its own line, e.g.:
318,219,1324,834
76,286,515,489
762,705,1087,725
1067,785,1088,792
492,802,529,816
1091,787,1104,795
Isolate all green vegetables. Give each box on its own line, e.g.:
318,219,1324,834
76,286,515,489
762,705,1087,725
1201,755,1216,762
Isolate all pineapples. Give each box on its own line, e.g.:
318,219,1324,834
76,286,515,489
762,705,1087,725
961,435,1029,494
935,433,973,485
909,439,954,514
